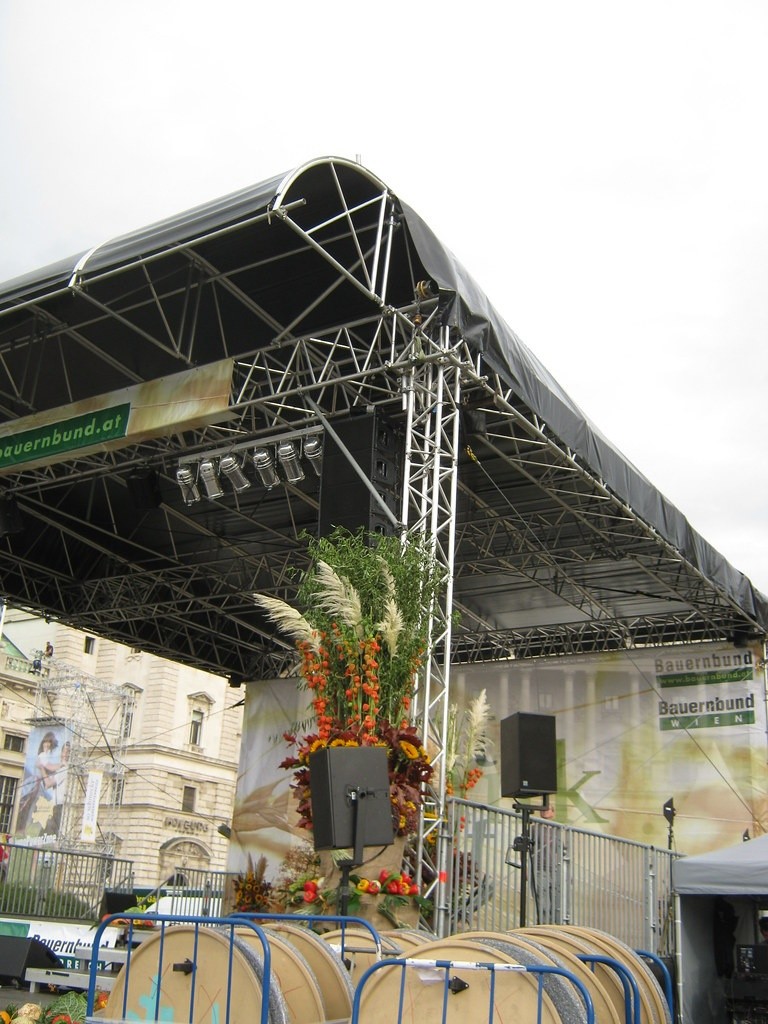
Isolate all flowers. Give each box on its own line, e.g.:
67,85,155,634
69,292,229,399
253,521,494,842
230,852,274,914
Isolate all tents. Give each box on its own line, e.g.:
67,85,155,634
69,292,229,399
671,834,768,1024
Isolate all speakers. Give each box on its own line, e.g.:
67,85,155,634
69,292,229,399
99,892,137,923
309,746,394,852
317,415,407,548
500,712,558,797
0,935,65,990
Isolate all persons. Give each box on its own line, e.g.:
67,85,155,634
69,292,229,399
19,733,70,819
527,804,568,925
44,642,53,657
760,916,768,948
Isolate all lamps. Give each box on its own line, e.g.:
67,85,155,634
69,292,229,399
176,436,322,505
